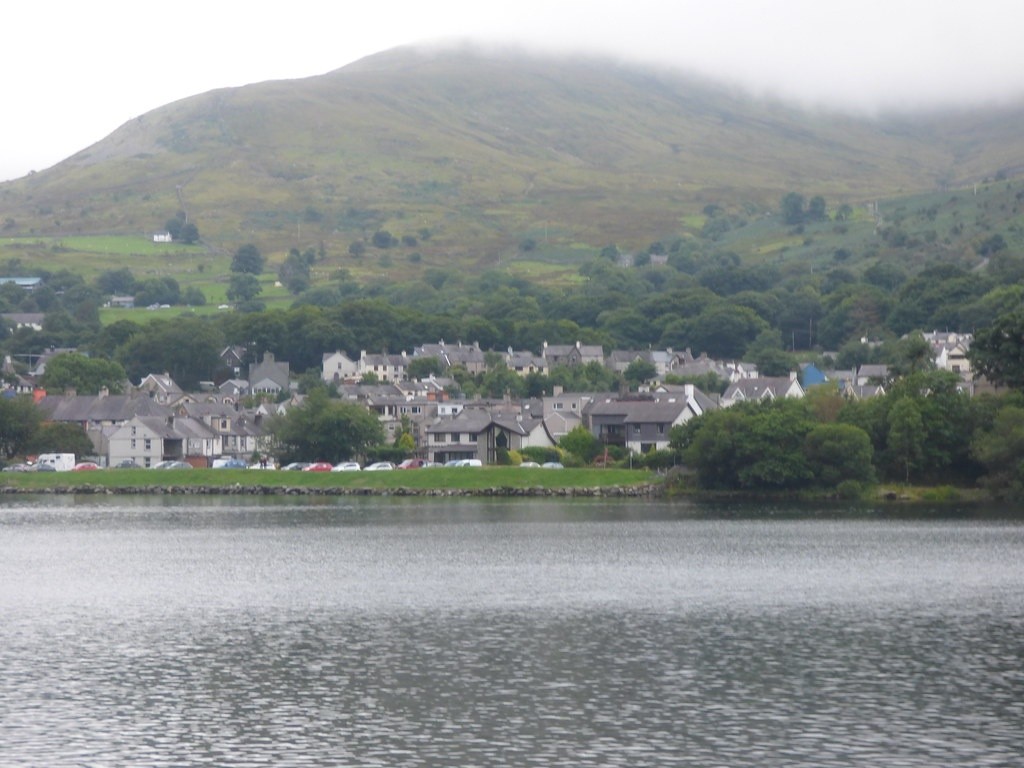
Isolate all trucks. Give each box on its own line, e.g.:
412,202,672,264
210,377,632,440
36,453,76,472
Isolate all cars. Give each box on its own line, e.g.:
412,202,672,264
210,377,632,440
165,460,194,471
27,462,57,472
218,458,248,469
519,462,564,470
362,461,397,472
303,462,334,472
395,458,427,471
422,458,482,468
71,462,98,471
280,461,307,472
2,463,26,473
112,459,142,470
146,460,174,470
331,461,361,472
